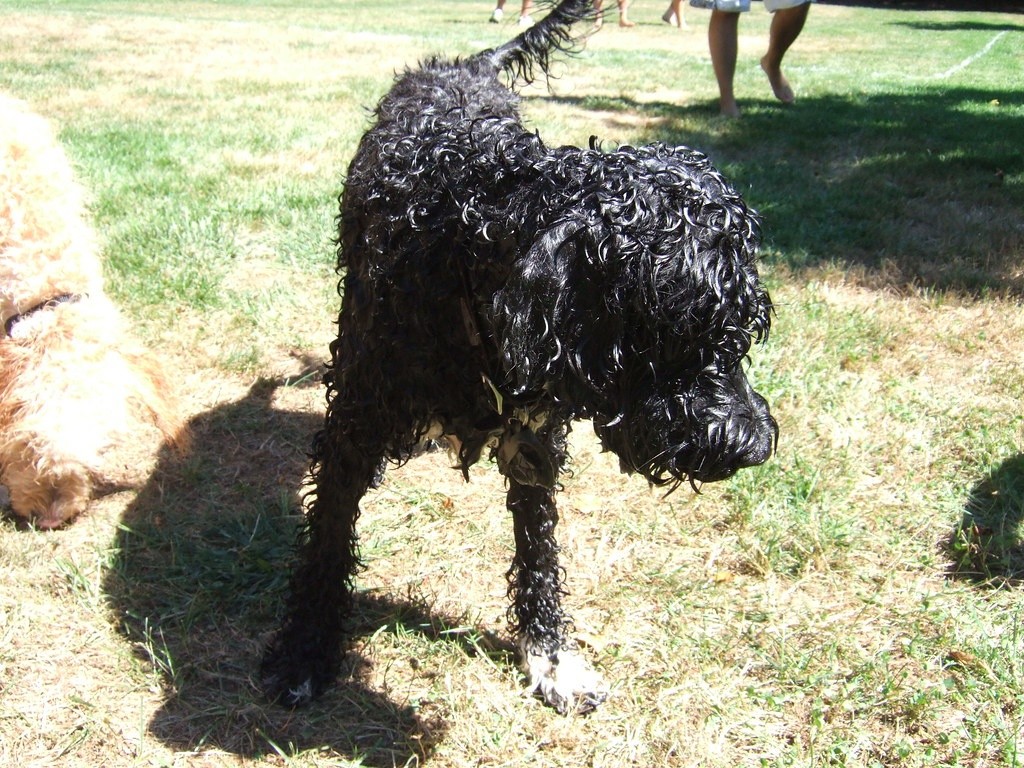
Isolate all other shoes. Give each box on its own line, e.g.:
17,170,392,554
489,9,503,23
663,14,677,26
518,16,535,27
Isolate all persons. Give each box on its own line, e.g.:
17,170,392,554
490,0,810,117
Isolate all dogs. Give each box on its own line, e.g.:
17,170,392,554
254,0,778,717
0,89,195,528
